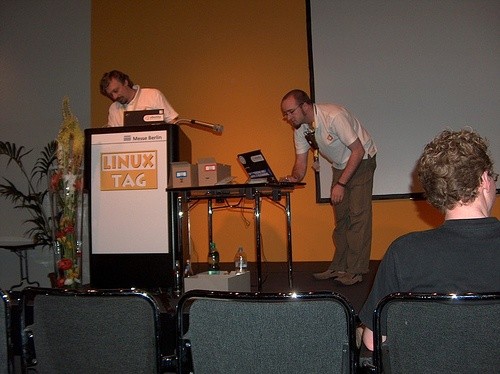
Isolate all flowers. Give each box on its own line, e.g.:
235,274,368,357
50,97,85,288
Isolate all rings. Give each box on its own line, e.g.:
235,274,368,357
286,177,287,179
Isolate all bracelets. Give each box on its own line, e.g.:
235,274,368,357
337,181,346,187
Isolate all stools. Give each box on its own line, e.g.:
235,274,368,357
0,236,40,288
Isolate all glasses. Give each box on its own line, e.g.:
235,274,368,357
282,102,305,121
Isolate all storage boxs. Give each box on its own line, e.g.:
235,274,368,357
169,162,198,187
197,162,231,186
184,269,251,293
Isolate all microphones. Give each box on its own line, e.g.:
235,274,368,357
185,119,223,133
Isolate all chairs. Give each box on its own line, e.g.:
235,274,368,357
0,290,500,374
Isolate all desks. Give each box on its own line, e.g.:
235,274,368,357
167,181,306,294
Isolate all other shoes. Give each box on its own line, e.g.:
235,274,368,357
334,272,363,286
312,270,346,280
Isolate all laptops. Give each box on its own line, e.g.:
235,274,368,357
237,150,306,184
123,109,164,126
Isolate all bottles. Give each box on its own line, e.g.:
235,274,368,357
185,260,191,278
208,242,219,276
235,247,248,273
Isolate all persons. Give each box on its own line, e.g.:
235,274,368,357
280,89,377,285
358,127,500,352
99,69,179,127
